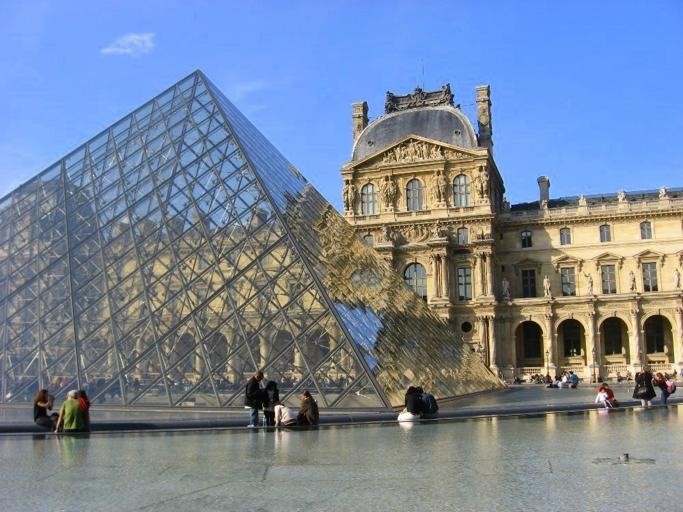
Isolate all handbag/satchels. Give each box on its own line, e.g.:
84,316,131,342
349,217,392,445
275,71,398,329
637,387,646,394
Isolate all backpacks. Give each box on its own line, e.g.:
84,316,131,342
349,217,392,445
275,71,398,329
665,381,675,394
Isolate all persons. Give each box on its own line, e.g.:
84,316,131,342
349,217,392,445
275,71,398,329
615,371,623,382
0,373,141,404
415,386,440,419
672,267,680,288
651,372,671,404
295,389,319,425
600,383,616,402
664,367,682,380
262,381,279,425
397,385,424,420
382,139,442,164
53,390,89,432
631,364,656,408
628,270,637,291
248,425,320,482
511,376,521,385
527,370,579,388
243,372,264,426
625,370,633,381
30,432,91,470
75,390,90,429
271,400,298,427
341,164,489,211
31,388,64,432
595,404,678,425
593,386,614,408
582,271,593,294
500,277,511,297
541,275,551,296
154,372,356,397
